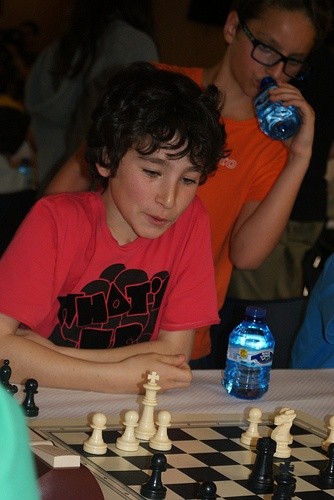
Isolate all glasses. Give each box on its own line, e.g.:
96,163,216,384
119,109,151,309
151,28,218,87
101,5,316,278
239,20,313,83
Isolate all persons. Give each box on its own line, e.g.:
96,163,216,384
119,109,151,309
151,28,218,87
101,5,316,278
0,0,334,500
0,62,233,396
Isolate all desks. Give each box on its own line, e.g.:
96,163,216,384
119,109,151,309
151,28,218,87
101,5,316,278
0,369,334,426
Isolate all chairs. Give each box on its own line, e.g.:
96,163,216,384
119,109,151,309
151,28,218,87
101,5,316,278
210,297,310,370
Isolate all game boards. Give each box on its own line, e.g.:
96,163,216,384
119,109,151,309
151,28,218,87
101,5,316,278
24,408,334,500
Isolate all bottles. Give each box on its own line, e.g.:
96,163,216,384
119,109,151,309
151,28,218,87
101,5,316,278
223,306,275,399
253,77,302,141
